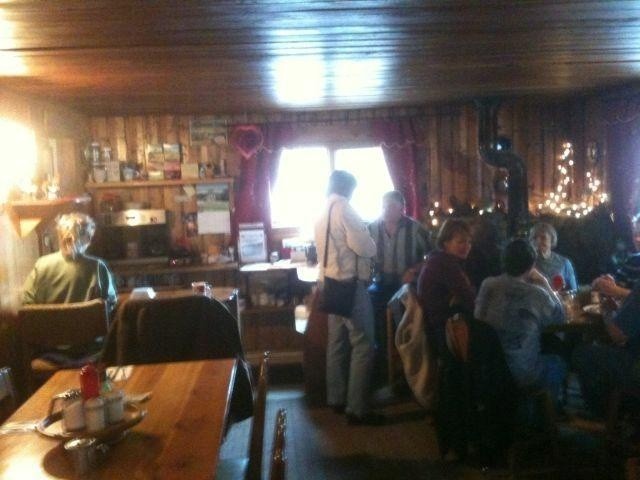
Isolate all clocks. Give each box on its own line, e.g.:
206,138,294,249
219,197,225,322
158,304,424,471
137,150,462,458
227,125,271,163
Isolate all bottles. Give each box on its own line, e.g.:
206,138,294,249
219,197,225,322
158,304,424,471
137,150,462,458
560,289,574,324
103,147,112,163
185,213,199,235
91,143,101,163
252,280,289,308
199,147,219,179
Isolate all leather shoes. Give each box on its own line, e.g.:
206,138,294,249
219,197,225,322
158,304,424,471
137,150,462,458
328,404,343,415
348,412,390,428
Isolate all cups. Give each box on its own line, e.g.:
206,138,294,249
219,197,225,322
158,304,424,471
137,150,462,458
560,289,580,321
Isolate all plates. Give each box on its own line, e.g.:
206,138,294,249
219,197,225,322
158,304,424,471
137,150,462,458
582,305,601,317
36,400,145,450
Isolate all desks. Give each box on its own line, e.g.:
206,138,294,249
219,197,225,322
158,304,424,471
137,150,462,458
0,355,238,480
116,282,241,329
529,285,615,335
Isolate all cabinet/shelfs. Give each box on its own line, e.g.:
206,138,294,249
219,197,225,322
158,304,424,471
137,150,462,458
107,252,297,349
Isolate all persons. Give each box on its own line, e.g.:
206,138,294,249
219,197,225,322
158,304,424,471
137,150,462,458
599,289,640,356
363,191,431,316
313,171,379,426
593,213,640,296
417,214,478,416
526,222,578,294
21,212,115,363
473,238,607,435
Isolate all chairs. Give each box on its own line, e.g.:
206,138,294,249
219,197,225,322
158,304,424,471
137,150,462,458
265,405,298,480
10,298,110,411
438,315,526,463
382,289,433,398
240,348,270,480
112,295,251,419
